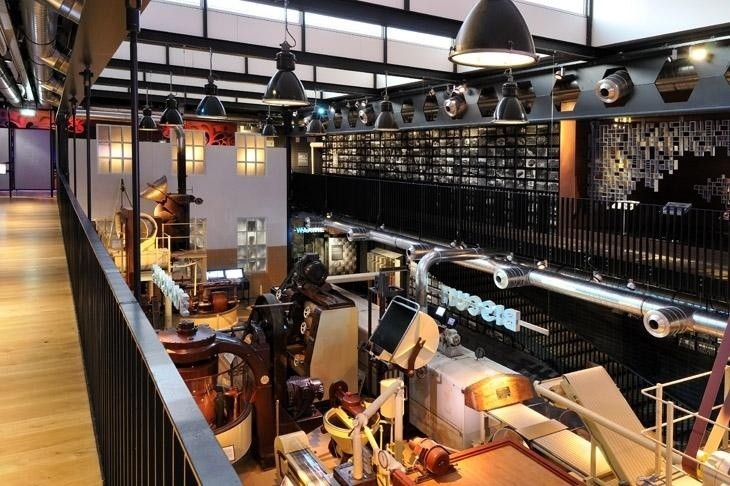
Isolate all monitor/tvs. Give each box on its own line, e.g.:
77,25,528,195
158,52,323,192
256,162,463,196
365,302,417,362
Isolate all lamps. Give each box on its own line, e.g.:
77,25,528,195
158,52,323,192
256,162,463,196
193,49,227,120
489,67,530,128
262,0,310,105
262,104,277,137
159,72,184,126
305,87,326,136
138,87,158,131
447,0,539,70
373,74,403,133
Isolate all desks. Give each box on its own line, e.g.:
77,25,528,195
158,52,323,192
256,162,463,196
211,278,250,305
